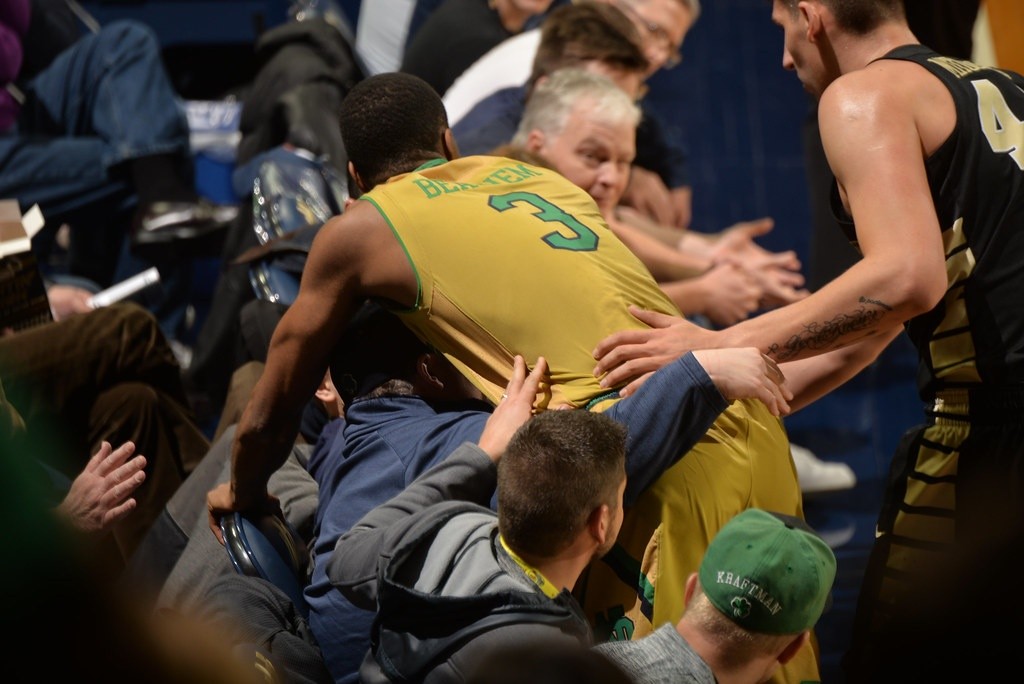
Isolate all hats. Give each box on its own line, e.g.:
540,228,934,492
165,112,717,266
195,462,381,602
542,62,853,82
698,508,837,637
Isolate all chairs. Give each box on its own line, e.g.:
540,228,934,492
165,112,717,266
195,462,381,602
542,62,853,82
254,195,319,246
253,157,334,223
287,0,370,78
188,82,345,399
219,509,308,618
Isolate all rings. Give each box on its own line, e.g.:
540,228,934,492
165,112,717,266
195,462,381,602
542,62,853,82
500,393,508,401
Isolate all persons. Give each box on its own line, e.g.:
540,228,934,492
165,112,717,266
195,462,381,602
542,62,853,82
595,0,1023,684
0,0,861,684
